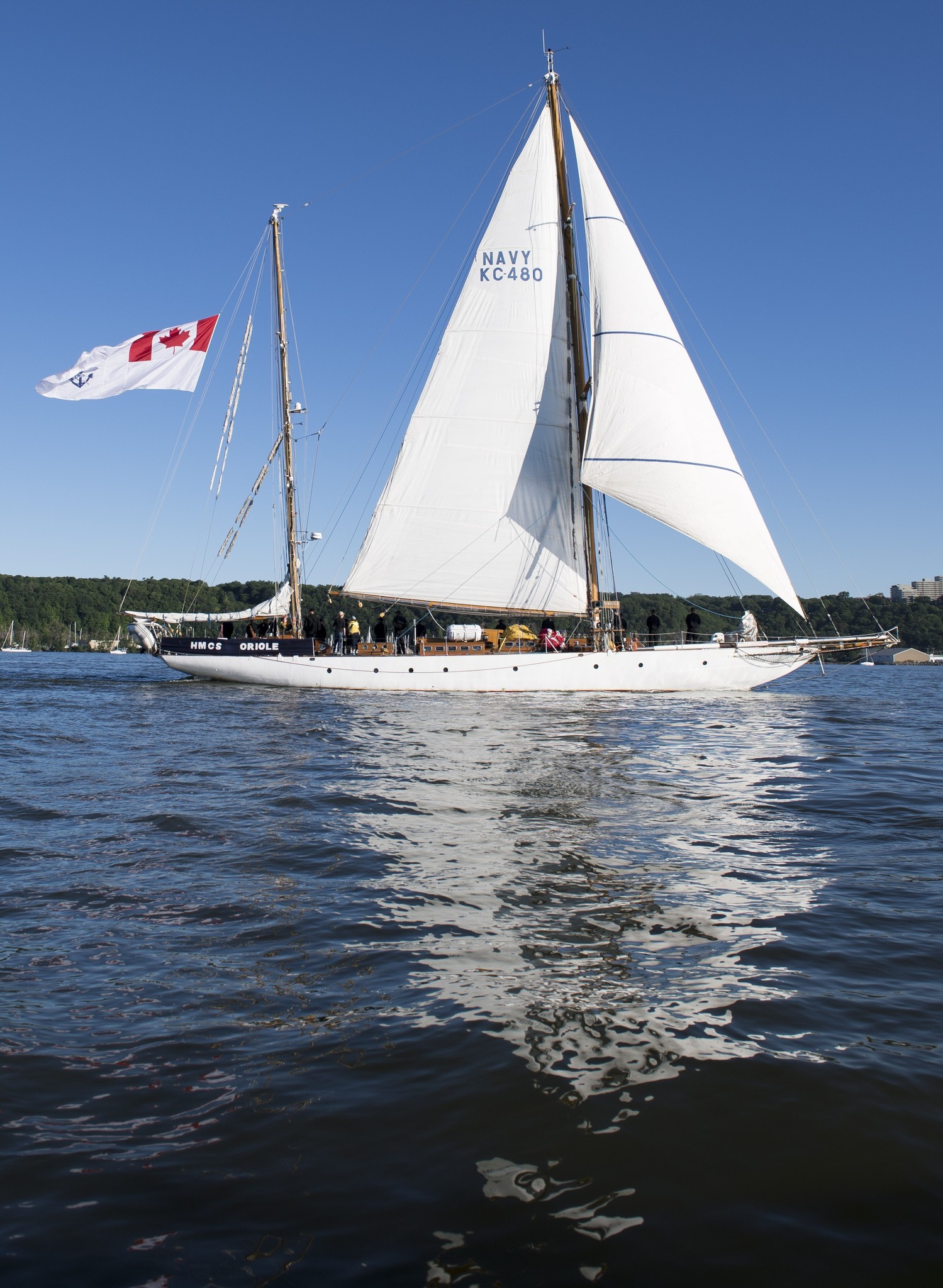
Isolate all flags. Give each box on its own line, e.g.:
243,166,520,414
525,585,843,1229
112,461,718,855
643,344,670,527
35,314,220,402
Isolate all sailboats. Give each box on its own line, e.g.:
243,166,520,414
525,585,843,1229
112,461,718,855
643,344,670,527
109,626,127,654
64,621,83,648
0,620,32,652
113,23,901,694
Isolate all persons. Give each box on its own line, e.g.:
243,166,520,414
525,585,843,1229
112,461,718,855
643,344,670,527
685,607,701,644
246,618,293,638
495,619,506,630
541,617,555,630
316,619,327,641
607,610,627,650
304,609,320,638
347,616,360,655
412,619,426,637
374,617,387,642
393,610,406,654
332,611,346,654
647,610,660,646
